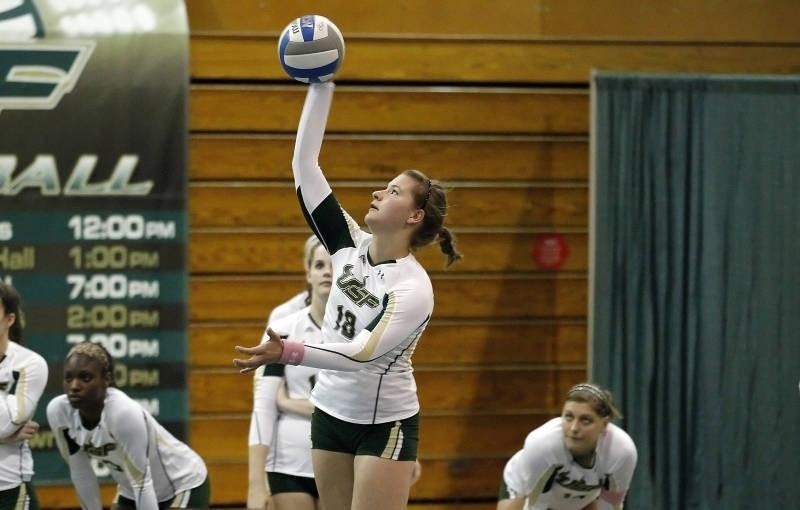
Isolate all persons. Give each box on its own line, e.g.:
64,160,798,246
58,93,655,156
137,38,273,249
247,240,421,510
496,383,638,510
253,234,320,510
0,281,50,510
233,79,463,510
47,342,211,510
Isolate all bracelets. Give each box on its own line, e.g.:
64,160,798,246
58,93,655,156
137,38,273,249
278,339,304,366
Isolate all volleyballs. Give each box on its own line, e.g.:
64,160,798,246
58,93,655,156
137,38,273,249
277,15,345,83
